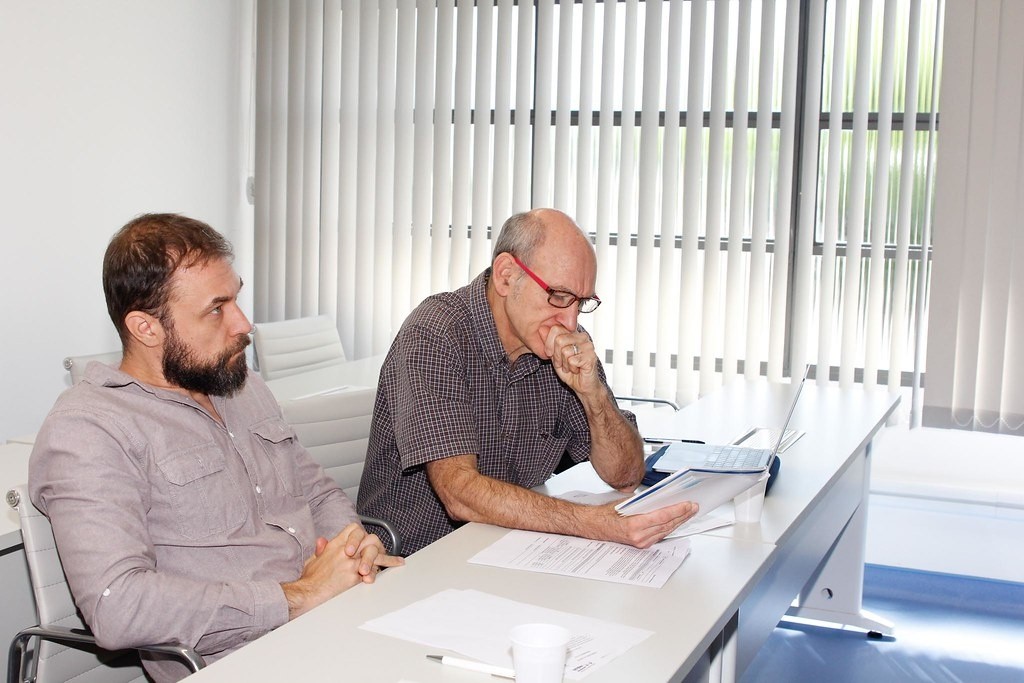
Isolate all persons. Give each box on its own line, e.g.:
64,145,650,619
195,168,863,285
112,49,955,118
28,214,404,683
357,208,699,571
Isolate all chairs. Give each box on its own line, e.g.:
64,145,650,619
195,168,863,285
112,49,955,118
280,387,400,555
63,352,125,378
0,487,204,683
250,311,346,384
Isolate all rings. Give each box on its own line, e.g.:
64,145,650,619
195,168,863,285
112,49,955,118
573,344,578,355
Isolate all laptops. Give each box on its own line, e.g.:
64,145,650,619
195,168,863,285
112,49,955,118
652,361,812,473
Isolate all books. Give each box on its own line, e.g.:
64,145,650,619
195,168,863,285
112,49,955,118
615,465,771,529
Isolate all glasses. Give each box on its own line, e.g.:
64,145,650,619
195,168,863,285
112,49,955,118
510,252,601,313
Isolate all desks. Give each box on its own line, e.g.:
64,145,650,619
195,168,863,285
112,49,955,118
184,380,902,683
